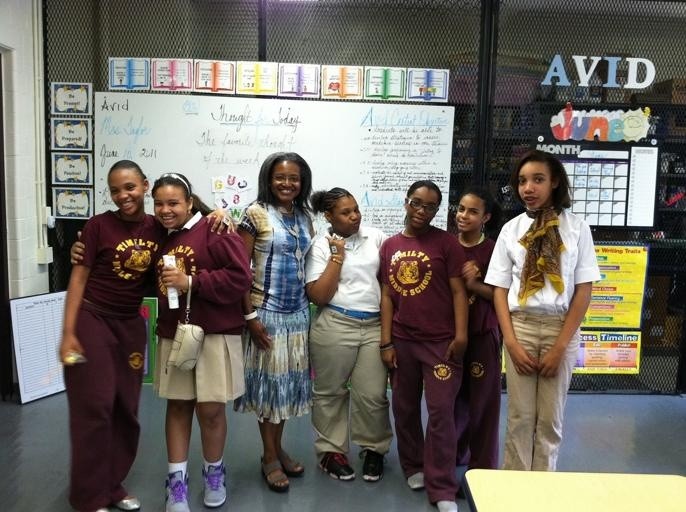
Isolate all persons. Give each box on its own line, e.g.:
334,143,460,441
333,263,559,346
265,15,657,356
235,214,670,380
59,160,236,512
304,187,390,482
234,153,317,493
70,172,252,512
483,149,601,471
449,184,503,498
378,180,471,512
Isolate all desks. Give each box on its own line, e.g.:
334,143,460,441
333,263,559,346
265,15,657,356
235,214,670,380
463,468,686,512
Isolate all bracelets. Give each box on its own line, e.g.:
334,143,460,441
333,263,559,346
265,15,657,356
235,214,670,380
244,310,258,322
331,254,343,265
378,341,396,351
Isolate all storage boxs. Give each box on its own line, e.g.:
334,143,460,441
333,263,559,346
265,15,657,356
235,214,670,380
630,92,659,102
654,78,685,104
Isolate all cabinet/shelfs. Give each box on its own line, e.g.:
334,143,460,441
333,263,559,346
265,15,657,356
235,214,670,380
450,102,686,246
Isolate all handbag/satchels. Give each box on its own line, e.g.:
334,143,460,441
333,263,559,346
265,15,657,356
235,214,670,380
166,320,203,371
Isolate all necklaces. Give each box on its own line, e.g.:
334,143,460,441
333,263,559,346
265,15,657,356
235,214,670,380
269,205,304,282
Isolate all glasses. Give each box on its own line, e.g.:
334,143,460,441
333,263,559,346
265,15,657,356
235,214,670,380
407,197,438,214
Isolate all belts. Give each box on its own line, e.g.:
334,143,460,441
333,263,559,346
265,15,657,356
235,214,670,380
328,304,379,319
